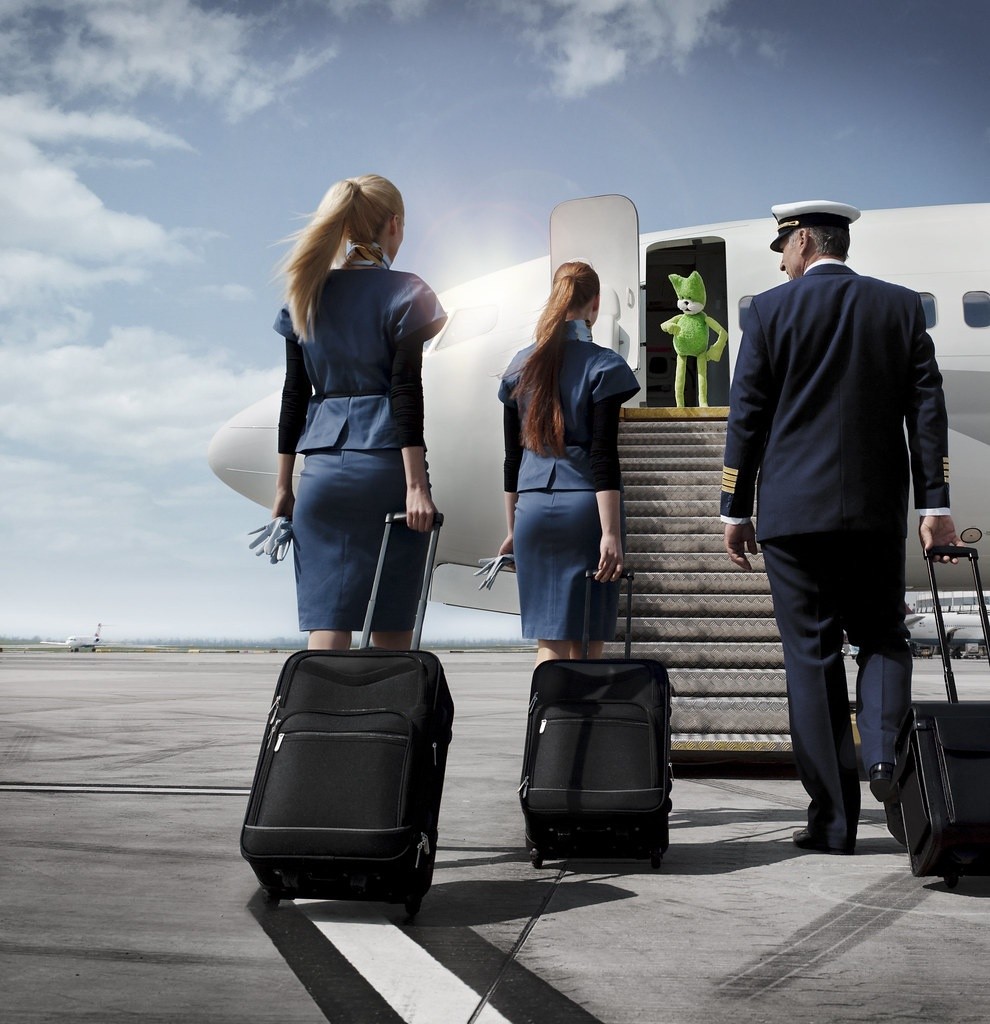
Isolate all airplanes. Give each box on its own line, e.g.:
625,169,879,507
904,612,990,659
208,193,990,766
40,623,117,652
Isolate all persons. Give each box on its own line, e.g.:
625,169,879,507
271,172,446,661
721,201,964,853
497,261,642,664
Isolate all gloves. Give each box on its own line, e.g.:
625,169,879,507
473,553,516,590
248,517,294,563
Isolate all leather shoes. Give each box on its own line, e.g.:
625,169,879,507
871,764,906,849
791,829,853,856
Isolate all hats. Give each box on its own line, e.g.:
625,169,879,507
769,199,861,252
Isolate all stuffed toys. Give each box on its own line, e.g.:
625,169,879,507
660,271,728,407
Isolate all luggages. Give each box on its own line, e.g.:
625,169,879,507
516,564,677,864
892,543,989,879
239,510,457,920
